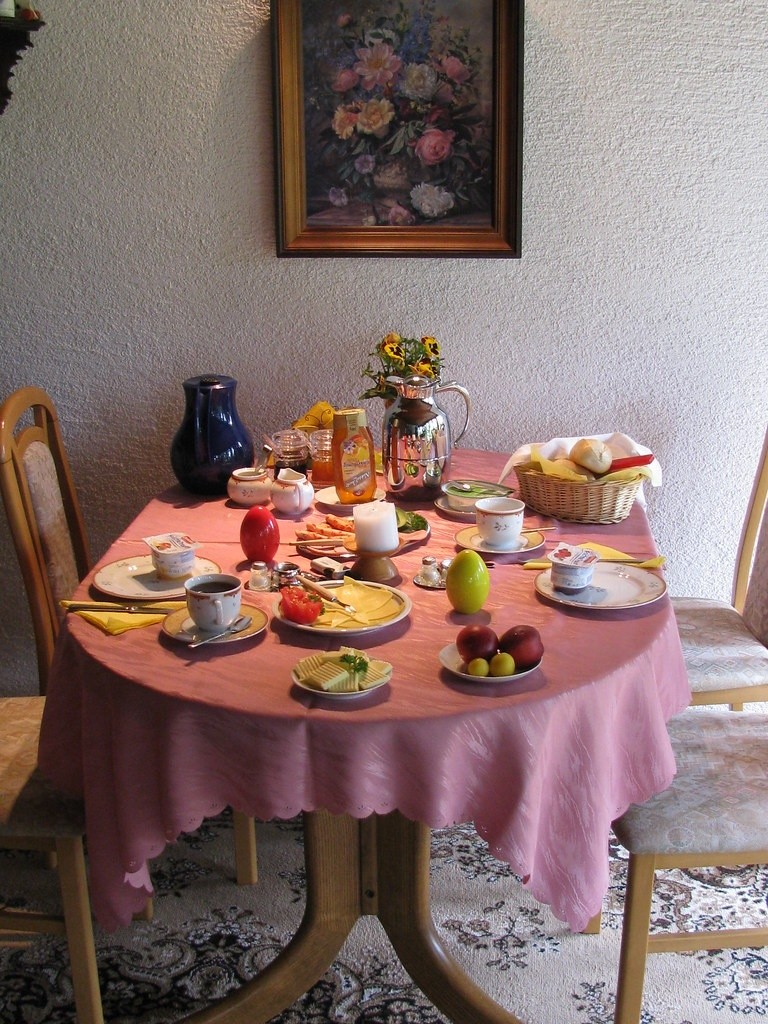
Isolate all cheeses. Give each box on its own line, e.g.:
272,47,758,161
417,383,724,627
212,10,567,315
294,645,393,692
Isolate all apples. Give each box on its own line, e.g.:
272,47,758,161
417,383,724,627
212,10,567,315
498,625,544,668
456,625,499,663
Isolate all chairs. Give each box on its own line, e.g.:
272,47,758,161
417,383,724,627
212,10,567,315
579,426,768,1024
0,389,256,1024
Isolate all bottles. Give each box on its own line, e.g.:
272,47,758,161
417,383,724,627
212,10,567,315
250,561,270,590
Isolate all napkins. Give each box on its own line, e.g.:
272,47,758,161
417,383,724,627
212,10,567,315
523,540,665,570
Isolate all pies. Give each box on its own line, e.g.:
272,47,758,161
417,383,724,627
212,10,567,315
293,513,355,541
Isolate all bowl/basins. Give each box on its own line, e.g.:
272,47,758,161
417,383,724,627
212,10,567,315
441,479,517,513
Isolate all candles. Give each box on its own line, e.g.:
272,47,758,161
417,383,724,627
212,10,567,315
352,501,399,551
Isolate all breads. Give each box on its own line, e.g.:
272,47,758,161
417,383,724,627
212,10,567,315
554,439,613,481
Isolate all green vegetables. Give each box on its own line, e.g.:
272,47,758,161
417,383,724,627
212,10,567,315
396,507,429,531
338,654,369,674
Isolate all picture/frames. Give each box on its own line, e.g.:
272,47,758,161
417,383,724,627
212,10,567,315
269,0,527,260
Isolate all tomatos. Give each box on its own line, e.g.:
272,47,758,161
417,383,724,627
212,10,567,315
279,586,320,625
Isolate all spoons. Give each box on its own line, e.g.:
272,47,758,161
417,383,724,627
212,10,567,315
188,616,253,648
448,479,508,496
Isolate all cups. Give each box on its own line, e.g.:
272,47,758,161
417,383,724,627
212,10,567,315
227,467,272,506
309,429,336,491
271,430,310,480
184,573,243,633
474,497,525,546
270,467,315,515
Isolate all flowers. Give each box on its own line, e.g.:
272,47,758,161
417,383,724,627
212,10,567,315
357,332,447,401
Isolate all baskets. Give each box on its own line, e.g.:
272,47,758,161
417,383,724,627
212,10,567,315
512,463,645,524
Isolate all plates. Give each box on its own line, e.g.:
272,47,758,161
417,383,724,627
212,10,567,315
439,642,543,683
314,485,386,513
533,562,668,610
271,579,413,637
161,603,269,644
453,524,547,554
296,515,431,558
433,495,476,522
291,656,393,700
91,553,223,599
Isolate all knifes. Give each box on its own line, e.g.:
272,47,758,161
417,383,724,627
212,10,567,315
523,558,645,570
68,604,174,614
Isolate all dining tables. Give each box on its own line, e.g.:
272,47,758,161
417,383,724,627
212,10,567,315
36,449,695,1024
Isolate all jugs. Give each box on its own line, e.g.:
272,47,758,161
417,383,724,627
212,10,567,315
170,374,256,498
382,374,472,503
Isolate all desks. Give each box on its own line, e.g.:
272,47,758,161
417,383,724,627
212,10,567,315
58,600,187,636
